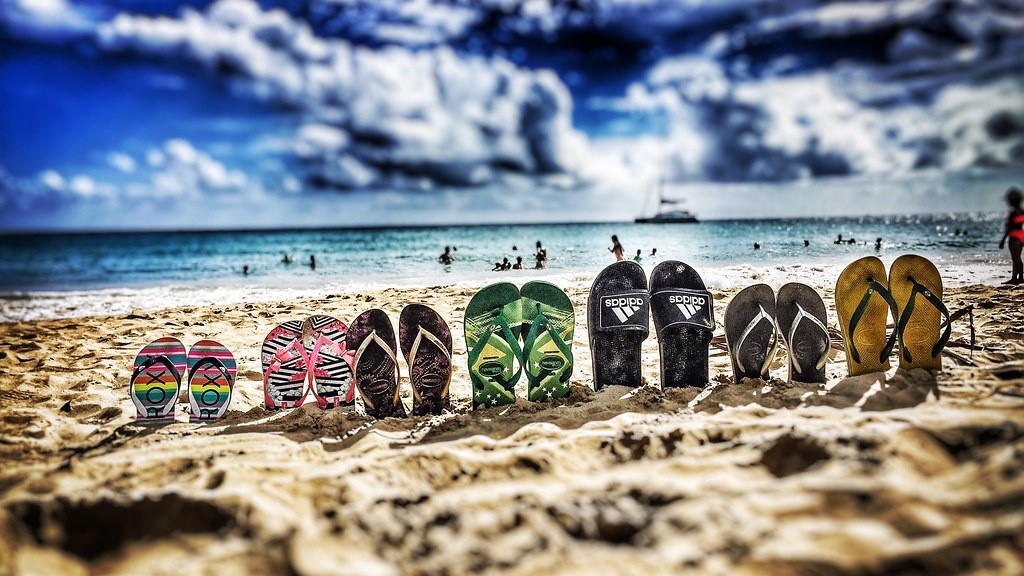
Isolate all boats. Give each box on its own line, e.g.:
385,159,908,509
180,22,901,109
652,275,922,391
634,210,700,224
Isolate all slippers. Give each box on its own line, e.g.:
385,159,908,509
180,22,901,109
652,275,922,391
345,309,408,420
131,337,187,425
464,282,522,411
520,280,575,402
188,340,236,422
723,283,776,385
262,320,308,408
776,282,831,385
303,316,355,408
586,260,650,389
888,254,952,370
835,256,891,377
398,304,453,415
649,263,716,390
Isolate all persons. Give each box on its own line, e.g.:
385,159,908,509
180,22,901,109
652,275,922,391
753,227,968,251
999,188,1024,286
242,252,316,274
440,235,657,270
